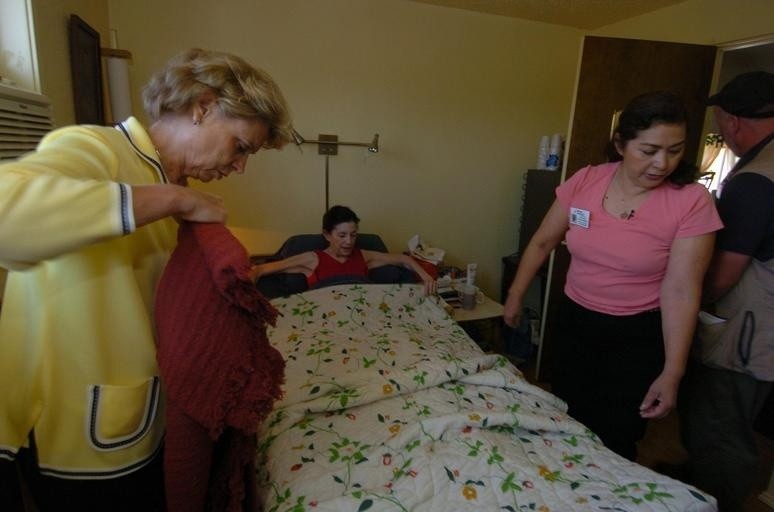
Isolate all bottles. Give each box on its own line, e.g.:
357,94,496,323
443,265,467,279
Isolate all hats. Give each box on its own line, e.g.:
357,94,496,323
705,72,770,119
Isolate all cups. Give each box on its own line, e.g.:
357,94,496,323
475,286,484,305
534,132,562,172
456,285,479,311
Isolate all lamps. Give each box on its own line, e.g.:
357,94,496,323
289,128,381,155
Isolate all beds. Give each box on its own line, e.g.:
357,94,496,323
233,227,724,512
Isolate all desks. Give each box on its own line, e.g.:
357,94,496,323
498,250,548,320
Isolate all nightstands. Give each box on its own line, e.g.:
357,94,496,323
451,290,507,353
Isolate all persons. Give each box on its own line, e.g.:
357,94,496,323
504,92,725,463
0,48,296,511
248,205,440,297
697,69,774,511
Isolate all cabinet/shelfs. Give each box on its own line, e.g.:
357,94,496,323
517,168,562,252
